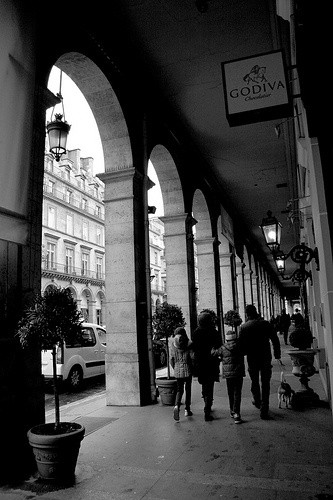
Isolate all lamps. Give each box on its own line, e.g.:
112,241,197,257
46,70,69,162
259,209,319,287
287,328,314,375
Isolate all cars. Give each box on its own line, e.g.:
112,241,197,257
41,322,107,393
153,338,167,367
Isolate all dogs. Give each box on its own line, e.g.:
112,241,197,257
275,370,294,409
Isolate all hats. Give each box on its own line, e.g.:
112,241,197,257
225,331,237,341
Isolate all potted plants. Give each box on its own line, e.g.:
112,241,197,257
150,304,185,406
224,310,242,341
17,287,86,482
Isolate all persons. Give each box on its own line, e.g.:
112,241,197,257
239,305,281,418
278,309,291,345
294,308,303,330
169,327,193,421
211,331,246,424
270,315,280,332
191,309,222,421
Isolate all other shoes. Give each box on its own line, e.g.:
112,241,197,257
261,412,268,420
185,410,192,416
230,411,233,417
252,401,260,408
173,406,179,420
205,415,213,421
234,413,241,423
